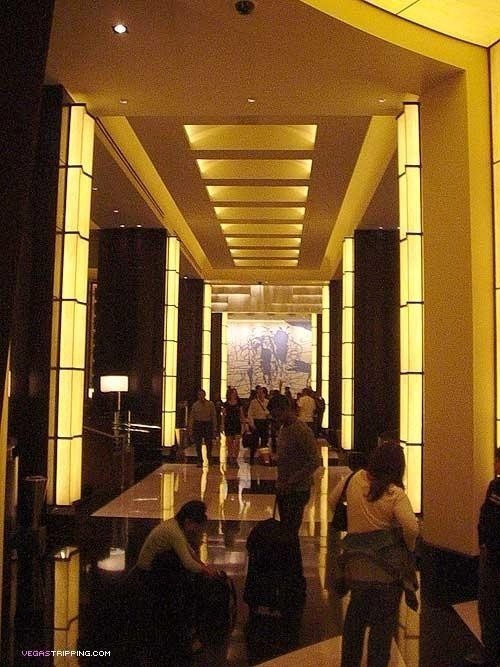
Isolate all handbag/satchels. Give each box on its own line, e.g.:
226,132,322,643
332,503,347,531
211,437,220,457
196,570,236,642
242,433,259,448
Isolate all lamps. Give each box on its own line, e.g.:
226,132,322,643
100,374,129,410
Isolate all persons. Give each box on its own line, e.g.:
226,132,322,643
245,327,289,392
263,396,322,600
138,499,237,645
328,442,420,666
187,384,324,468
464,447,499,664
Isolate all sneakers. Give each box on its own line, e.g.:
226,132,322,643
465,652,486,663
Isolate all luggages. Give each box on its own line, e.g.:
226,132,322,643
243,496,307,619
